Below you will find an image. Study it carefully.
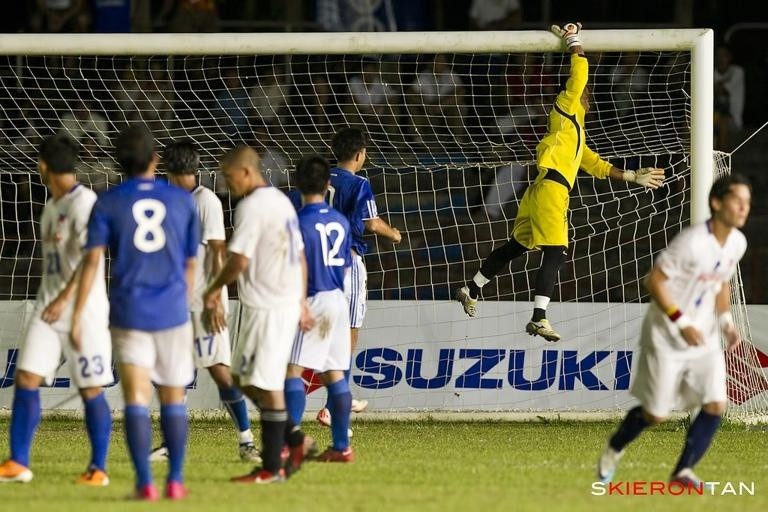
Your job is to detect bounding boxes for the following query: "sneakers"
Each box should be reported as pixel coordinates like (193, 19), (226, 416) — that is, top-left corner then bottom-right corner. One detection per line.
(316, 407), (354, 438)
(0, 459), (34, 484)
(524, 317), (562, 343)
(455, 285), (481, 318)
(667, 469), (709, 490)
(596, 438), (625, 486)
(350, 398), (369, 413)
(238, 441), (264, 465)
(230, 469), (281, 486)
(163, 480), (191, 502)
(280, 432), (317, 480)
(72, 468), (109, 487)
(314, 446), (354, 462)
(148, 446), (170, 462)
(126, 481), (159, 503)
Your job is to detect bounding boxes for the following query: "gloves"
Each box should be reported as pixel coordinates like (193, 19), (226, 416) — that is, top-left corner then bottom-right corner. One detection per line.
(550, 21), (584, 49)
(622, 165), (669, 191)
